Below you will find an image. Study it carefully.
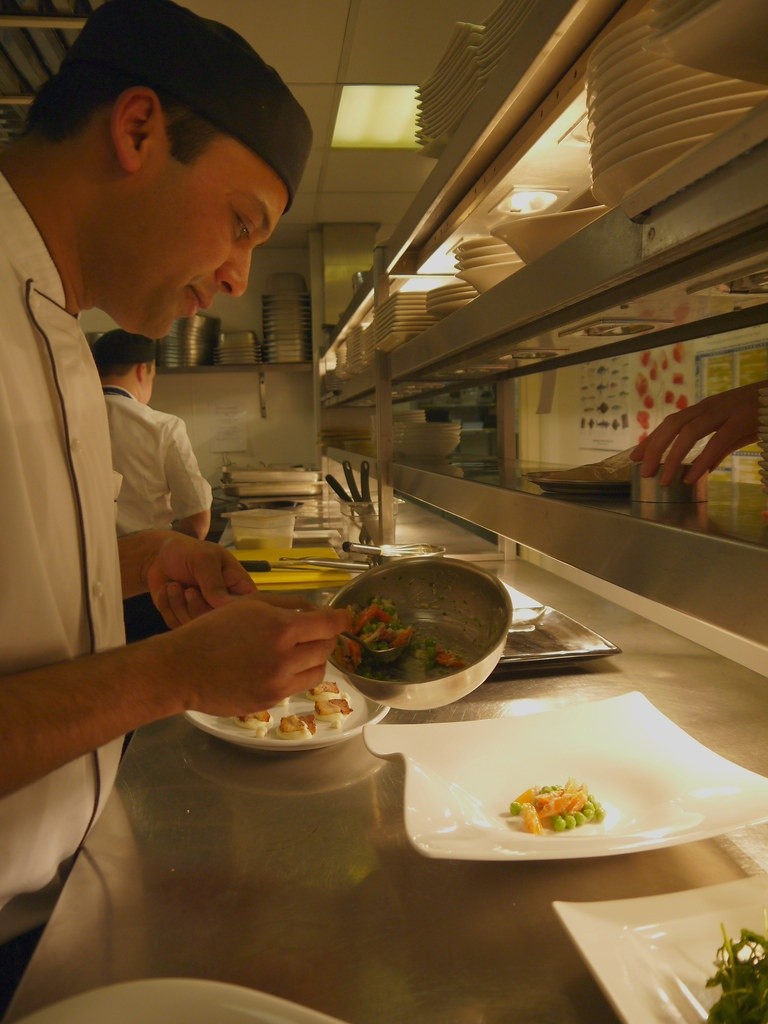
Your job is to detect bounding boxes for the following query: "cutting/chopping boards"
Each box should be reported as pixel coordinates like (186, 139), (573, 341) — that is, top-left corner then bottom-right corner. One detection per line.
(226, 548), (353, 583)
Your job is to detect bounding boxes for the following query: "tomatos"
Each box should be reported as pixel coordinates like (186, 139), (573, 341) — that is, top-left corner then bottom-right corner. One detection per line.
(334, 604), (467, 668)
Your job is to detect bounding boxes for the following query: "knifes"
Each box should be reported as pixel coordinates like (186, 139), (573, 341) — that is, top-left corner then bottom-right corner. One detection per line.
(324, 459), (379, 546)
(239, 560), (307, 572)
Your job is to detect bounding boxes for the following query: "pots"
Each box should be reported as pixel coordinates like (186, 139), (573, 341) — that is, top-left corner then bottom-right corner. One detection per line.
(279, 543), (447, 567)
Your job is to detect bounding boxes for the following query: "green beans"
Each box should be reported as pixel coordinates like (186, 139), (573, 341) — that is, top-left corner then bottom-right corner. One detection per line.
(356, 595), (438, 678)
(510, 786), (606, 831)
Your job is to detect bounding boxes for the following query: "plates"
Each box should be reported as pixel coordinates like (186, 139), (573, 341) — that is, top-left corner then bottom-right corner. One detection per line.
(12, 976), (351, 1024)
(498, 606), (624, 667)
(184, 659), (390, 752)
(503, 581), (544, 609)
(322, 0), (768, 405)
(240, 500), (304, 511)
(525, 470), (633, 494)
(364, 691), (768, 860)
(553, 872), (768, 1024)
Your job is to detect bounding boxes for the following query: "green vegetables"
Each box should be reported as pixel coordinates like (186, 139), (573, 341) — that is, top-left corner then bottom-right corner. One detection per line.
(703, 908), (768, 1024)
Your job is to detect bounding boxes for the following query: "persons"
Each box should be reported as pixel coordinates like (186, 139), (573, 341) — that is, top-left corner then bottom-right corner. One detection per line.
(630, 378), (768, 487)
(0, 0), (354, 1024)
(91, 329), (213, 646)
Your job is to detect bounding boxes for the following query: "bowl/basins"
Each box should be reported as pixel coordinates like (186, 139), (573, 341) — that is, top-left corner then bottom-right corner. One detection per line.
(328, 556), (512, 710)
(370, 409), (497, 461)
(155, 270), (314, 370)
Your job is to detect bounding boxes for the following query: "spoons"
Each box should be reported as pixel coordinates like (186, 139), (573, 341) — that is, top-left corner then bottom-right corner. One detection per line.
(213, 496), (247, 511)
(295, 608), (408, 662)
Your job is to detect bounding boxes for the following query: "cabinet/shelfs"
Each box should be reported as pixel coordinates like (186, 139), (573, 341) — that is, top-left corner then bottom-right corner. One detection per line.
(317, 0), (768, 677)
